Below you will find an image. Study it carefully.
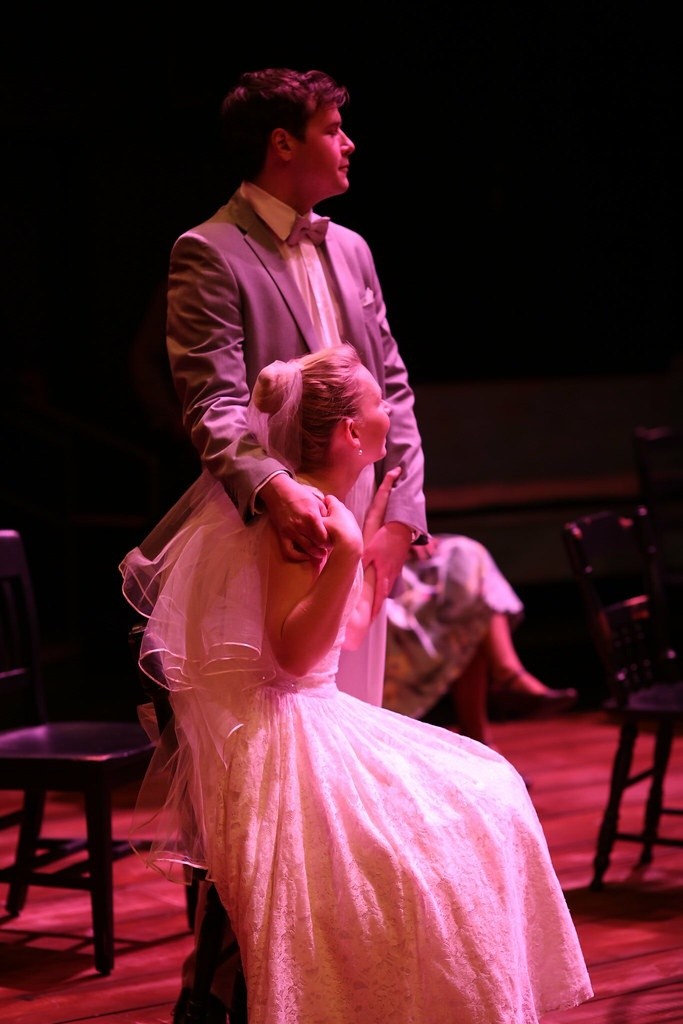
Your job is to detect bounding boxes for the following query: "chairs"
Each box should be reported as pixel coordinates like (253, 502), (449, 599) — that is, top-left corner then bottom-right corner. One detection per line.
(561, 503), (683, 878)
(629, 424), (683, 669)
(127, 620), (247, 1024)
(0, 529), (198, 972)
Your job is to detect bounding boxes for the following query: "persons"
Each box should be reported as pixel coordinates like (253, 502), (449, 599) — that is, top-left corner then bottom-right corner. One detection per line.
(167, 70), (426, 1023)
(115, 340), (595, 1024)
(382, 532), (578, 784)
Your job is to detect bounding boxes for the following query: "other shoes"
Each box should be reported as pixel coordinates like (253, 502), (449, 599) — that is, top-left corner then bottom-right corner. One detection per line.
(486, 675), (575, 721)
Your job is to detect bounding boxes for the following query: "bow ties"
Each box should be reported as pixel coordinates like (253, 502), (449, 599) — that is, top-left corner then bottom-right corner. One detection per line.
(285, 217), (329, 250)
